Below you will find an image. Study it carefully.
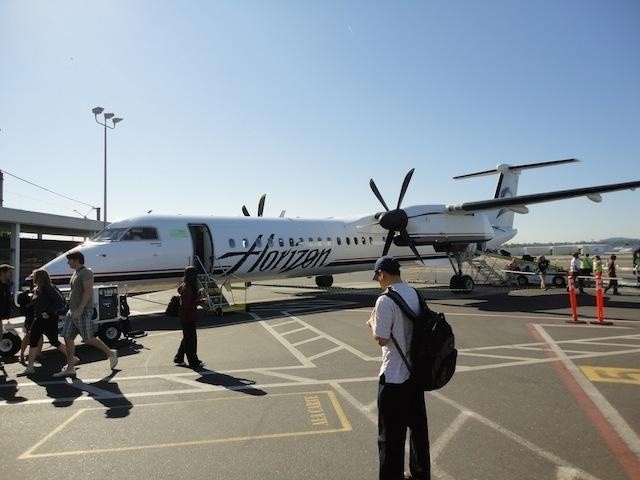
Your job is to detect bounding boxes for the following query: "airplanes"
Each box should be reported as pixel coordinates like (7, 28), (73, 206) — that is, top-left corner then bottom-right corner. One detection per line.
(23, 155), (640, 311)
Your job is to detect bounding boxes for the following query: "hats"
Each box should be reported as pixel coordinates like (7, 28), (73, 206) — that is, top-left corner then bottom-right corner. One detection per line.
(372, 256), (401, 281)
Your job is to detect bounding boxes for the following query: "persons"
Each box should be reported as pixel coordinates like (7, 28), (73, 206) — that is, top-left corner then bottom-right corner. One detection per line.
(507, 258), (520, 289)
(594, 255), (603, 273)
(17, 268), (81, 378)
(17, 274), (48, 366)
(365, 254), (432, 480)
(0, 263), (17, 371)
(172, 265), (207, 368)
(51, 249), (118, 379)
(631, 250), (640, 288)
(566, 252), (585, 293)
(537, 254), (551, 289)
(583, 253), (593, 288)
(604, 254), (621, 295)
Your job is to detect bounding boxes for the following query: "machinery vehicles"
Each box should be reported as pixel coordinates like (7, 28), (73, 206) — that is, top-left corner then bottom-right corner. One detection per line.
(502, 258), (574, 286)
(1, 283), (147, 357)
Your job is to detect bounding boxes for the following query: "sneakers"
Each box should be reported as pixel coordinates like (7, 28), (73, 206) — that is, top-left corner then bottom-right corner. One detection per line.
(539, 288), (622, 296)
(109, 349), (118, 370)
(404, 469), (413, 479)
(173, 358), (205, 369)
(16, 357), (80, 379)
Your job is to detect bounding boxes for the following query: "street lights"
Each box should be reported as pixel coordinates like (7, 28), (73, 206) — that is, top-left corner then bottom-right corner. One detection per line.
(92, 106), (123, 229)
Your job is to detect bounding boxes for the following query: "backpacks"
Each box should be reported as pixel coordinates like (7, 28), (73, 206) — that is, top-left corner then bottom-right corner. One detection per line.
(38, 285), (65, 308)
(374, 286), (458, 392)
